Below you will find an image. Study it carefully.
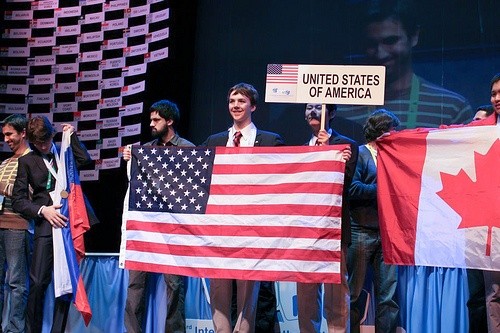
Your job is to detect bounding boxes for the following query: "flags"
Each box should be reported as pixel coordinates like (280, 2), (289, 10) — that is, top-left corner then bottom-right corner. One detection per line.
(49, 128), (93, 328)
(377, 124), (500, 271)
(266, 64), (298, 83)
(117, 144), (349, 286)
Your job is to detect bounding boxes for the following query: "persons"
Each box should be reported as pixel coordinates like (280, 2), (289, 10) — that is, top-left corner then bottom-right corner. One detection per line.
(122, 100), (197, 333)
(485, 79), (500, 331)
(472, 105), (493, 123)
(197, 83), (297, 333)
(351, 109), (404, 333)
(298, 104), (359, 333)
(10, 113), (100, 333)
(336, 0), (473, 135)
(0, 114), (33, 333)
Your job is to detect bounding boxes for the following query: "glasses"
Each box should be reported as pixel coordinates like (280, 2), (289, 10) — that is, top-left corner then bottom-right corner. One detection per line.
(33, 138), (51, 144)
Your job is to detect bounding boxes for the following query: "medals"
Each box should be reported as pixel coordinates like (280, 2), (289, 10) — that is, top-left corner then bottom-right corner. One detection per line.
(60, 190), (69, 199)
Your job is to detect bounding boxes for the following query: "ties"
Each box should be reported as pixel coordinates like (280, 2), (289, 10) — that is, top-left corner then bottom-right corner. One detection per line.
(232, 131), (243, 147)
(315, 139), (322, 146)
